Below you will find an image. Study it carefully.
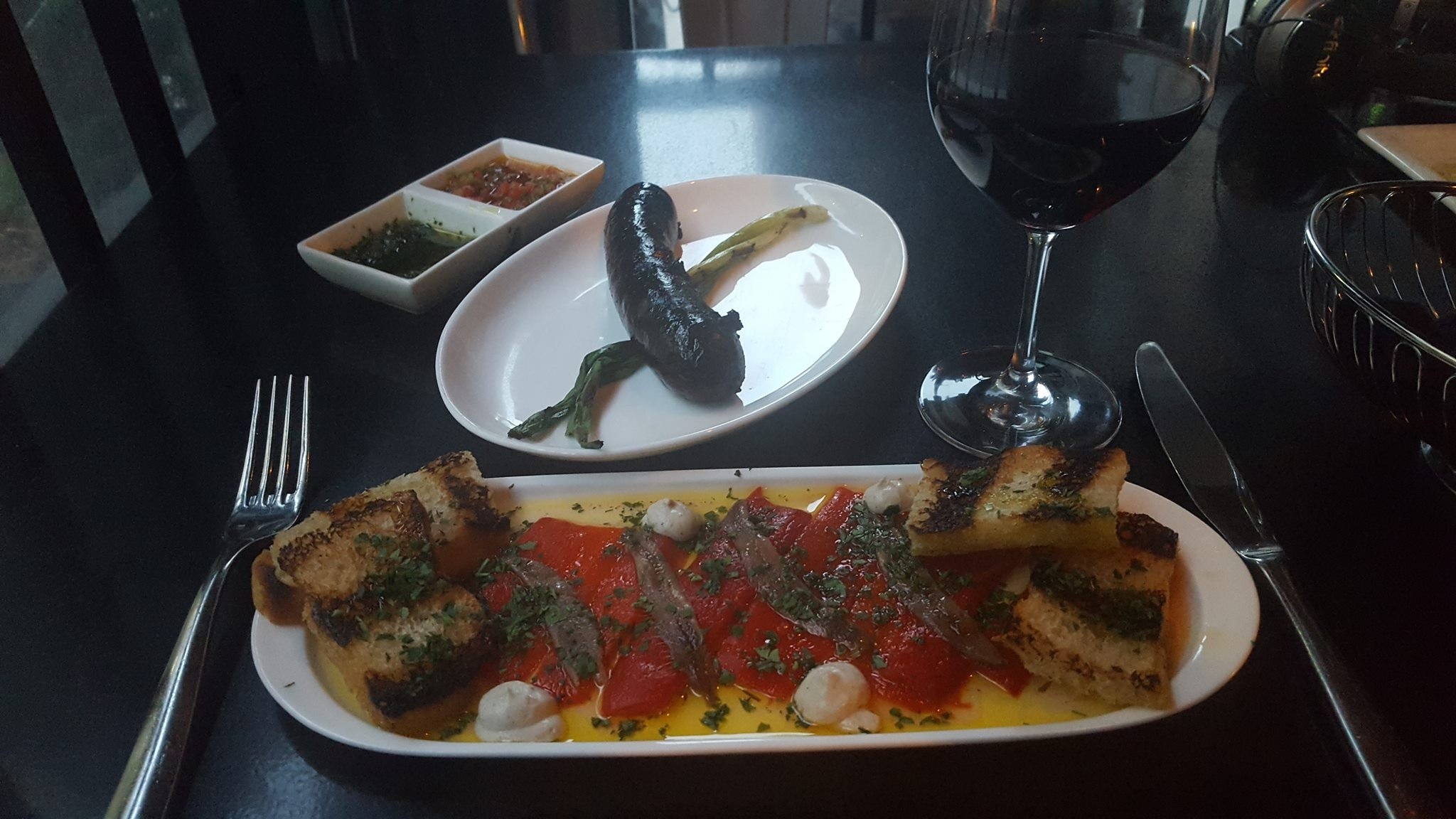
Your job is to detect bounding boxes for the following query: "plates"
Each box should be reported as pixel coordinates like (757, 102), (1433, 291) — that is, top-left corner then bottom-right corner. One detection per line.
(247, 458), (1263, 760)
(434, 172), (905, 461)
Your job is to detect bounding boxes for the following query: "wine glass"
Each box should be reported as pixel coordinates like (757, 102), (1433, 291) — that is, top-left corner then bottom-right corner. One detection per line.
(917, 0), (1230, 460)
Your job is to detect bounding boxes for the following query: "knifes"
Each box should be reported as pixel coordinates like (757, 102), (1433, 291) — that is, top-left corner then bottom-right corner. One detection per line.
(1133, 337), (1450, 819)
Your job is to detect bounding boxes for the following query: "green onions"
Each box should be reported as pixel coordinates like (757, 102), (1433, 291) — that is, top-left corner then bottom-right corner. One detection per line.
(506, 202), (829, 447)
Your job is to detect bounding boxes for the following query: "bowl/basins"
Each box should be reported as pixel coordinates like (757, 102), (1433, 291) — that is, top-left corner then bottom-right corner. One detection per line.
(298, 136), (607, 316)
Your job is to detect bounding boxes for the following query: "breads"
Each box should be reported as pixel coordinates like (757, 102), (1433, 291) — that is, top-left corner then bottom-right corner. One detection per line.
(244, 448), (509, 630)
(895, 440), (1127, 566)
(296, 600), (534, 746)
(1007, 512), (1179, 712)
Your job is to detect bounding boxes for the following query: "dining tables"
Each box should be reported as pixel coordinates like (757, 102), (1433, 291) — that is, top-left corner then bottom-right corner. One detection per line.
(0, 49), (1456, 819)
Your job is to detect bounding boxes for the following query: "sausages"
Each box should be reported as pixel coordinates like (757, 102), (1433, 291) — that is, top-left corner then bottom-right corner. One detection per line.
(604, 176), (748, 402)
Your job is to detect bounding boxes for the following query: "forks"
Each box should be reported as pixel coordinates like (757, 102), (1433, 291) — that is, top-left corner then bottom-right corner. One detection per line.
(95, 369), (312, 819)
